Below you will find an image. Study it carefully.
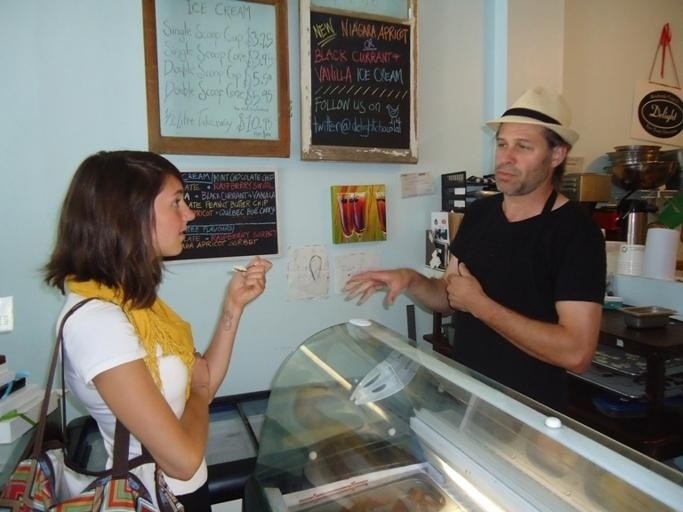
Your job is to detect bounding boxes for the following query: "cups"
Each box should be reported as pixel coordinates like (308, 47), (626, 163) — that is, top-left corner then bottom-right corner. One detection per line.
(337, 193), (387, 242)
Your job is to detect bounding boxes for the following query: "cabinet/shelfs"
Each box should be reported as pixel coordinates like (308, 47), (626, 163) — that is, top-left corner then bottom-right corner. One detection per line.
(243, 320), (683, 512)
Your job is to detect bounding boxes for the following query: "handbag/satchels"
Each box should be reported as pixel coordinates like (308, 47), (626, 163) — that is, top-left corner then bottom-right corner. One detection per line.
(0, 440), (185, 512)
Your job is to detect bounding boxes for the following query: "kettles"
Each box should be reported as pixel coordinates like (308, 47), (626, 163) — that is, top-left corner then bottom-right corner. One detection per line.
(617, 189), (657, 246)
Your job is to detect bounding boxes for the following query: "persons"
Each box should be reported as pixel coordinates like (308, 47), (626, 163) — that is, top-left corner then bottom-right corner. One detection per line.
(40, 149), (274, 512)
(340, 86), (607, 432)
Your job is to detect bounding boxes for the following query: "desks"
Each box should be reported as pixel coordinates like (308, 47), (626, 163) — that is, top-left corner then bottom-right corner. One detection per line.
(423, 305), (683, 463)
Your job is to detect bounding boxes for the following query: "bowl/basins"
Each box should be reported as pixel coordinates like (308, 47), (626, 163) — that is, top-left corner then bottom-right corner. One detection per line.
(603, 146), (681, 190)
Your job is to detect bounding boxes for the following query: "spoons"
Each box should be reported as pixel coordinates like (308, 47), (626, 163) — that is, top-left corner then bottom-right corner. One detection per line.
(233, 264), (256, 273)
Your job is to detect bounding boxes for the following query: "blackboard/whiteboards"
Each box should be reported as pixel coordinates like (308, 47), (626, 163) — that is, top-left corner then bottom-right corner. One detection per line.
(162, 172), (278, 262)
(301, 6), (417, 163)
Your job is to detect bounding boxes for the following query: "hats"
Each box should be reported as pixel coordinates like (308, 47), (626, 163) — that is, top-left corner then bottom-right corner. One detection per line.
(485, 88), (580, 147)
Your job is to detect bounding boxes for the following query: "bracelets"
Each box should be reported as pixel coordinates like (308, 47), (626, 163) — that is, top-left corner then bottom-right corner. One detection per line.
(189, 381), (212, 392)
